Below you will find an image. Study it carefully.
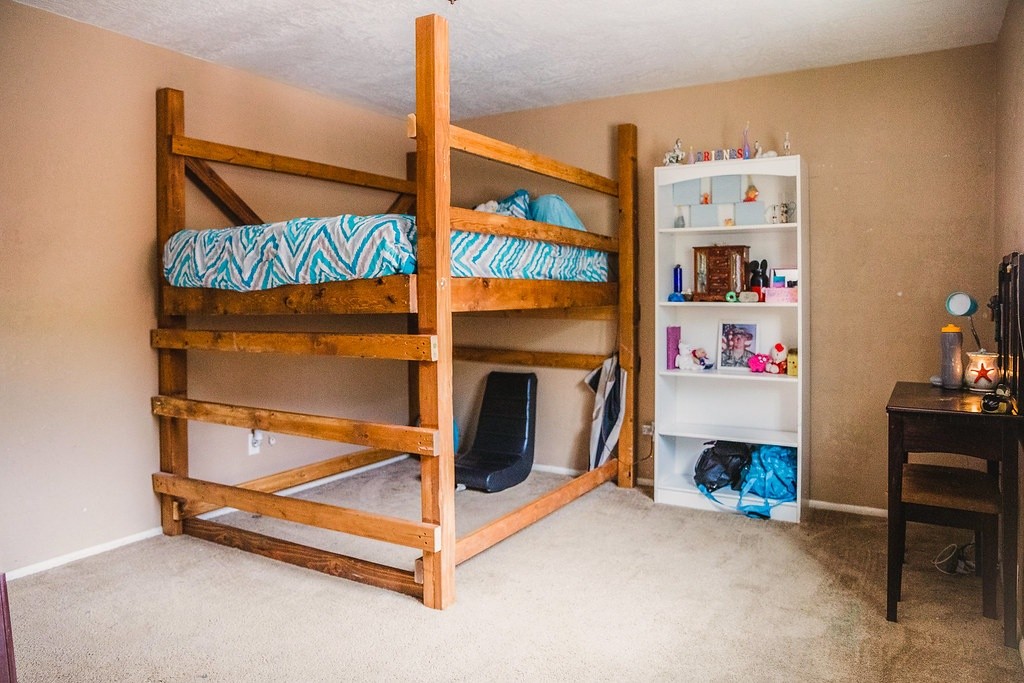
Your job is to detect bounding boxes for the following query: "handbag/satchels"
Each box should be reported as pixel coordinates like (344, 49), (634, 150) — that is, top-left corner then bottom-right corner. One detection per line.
(742, 445), (798, 501)
(694, 440), (752, 492)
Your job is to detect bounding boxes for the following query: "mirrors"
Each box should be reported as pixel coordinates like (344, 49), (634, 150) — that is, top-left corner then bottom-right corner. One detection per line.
(770, 267), (798, 288)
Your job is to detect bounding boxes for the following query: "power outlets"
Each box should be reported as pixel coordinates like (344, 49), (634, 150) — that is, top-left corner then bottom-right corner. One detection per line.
(643, 425), (654, 435)
(250, 426), (261, 454)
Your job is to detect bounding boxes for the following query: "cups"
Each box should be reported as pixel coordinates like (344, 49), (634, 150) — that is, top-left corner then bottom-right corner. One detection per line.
(770, 201), (796, 224)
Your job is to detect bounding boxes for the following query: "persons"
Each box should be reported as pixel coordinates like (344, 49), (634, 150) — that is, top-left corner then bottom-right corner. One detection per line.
(721, 328), (755, 369)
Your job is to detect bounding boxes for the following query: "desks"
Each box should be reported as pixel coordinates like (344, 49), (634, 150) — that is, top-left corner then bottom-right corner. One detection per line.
(885, 379), (1021, 649)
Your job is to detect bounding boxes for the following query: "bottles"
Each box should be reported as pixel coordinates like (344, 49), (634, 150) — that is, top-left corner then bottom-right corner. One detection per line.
(941, 324), (964, 389)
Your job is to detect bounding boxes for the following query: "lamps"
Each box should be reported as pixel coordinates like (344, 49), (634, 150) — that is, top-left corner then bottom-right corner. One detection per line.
(945, 290), (983, 348)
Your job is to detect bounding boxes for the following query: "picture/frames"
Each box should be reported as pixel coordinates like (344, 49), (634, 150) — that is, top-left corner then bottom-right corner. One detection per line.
(717, 321), (762, 369)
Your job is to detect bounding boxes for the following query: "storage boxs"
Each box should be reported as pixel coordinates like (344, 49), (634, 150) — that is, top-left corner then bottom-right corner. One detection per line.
(765, 287), (797, 303)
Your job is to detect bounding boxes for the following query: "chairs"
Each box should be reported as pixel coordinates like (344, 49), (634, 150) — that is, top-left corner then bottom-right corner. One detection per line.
(455, 372), (539, 493)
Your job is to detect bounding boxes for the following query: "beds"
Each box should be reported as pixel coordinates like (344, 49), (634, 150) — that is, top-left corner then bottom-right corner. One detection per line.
(156, 10), (636, 608)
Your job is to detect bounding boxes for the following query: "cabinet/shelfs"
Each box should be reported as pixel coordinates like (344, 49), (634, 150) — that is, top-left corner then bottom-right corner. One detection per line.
(644, 156), (811, 524)
